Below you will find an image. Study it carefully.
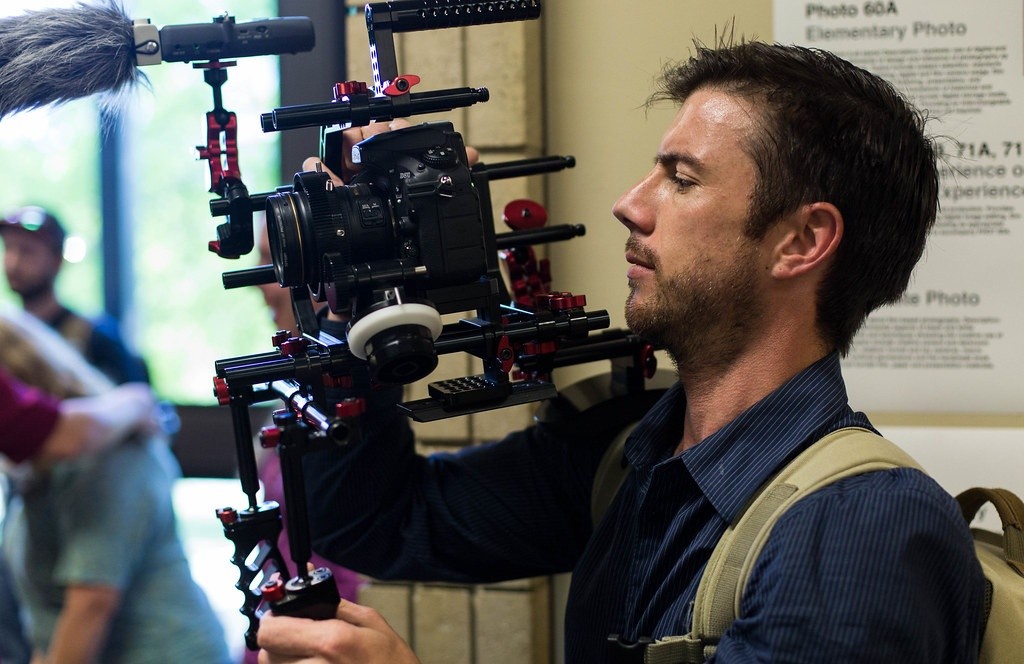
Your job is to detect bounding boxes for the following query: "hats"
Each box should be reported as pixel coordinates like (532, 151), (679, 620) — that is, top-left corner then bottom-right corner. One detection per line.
(0, 205), (64, 255)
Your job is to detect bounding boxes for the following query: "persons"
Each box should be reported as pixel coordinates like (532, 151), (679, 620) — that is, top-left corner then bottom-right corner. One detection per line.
(1, 203), (232, 664)
(239, 215), (358, 664)
(256, 37), (986, 664)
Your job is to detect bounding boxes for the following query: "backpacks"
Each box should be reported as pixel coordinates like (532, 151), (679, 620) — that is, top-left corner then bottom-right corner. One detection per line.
(591, 415), (1024, 664)
